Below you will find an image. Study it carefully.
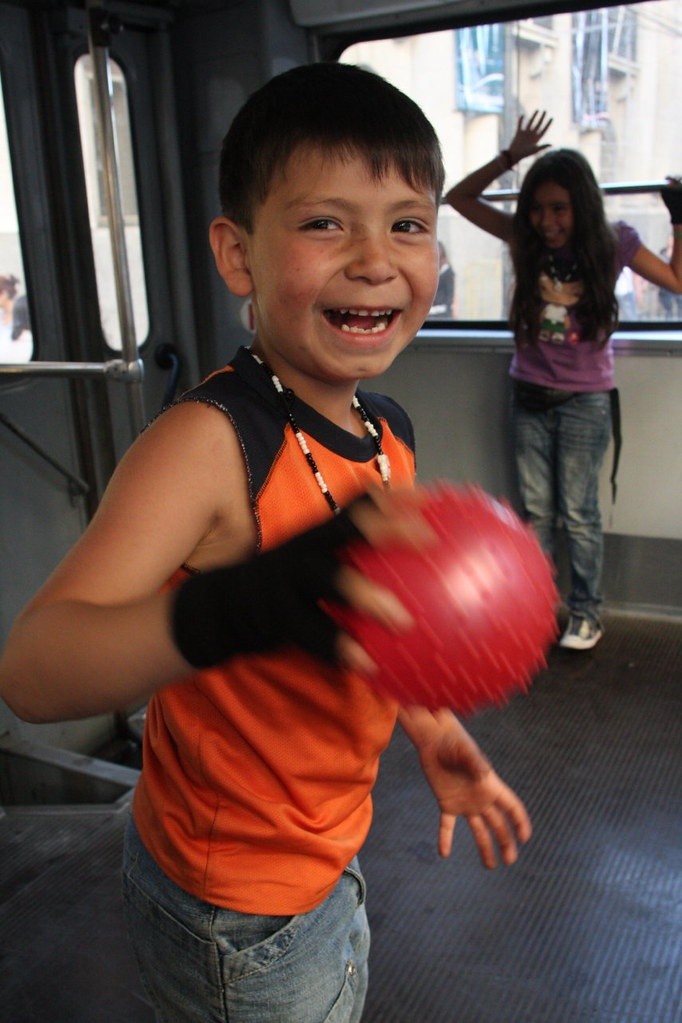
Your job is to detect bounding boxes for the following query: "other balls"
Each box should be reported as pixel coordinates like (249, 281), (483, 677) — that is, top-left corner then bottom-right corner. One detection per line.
(345, 480), (563, 712)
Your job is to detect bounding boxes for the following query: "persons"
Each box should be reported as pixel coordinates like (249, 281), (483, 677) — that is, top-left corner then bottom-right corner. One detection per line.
(0, 274), (36, 360)
(0, 58), (536, 1022)
(445, 107), (682, 652)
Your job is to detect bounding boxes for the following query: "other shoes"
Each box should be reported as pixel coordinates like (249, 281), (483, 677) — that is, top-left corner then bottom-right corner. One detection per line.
(561, 611), (603, 648)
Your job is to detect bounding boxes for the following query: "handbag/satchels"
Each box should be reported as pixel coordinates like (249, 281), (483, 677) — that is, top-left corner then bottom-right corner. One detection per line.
(515, 380), (575, 412)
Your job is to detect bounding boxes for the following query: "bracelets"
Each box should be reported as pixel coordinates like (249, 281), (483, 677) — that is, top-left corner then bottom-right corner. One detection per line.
(499, 150), (513, 170)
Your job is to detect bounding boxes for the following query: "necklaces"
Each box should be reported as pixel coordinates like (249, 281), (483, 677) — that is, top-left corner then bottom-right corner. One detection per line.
(243, 344), (393, 517)
(548, 252), (577, 292)
